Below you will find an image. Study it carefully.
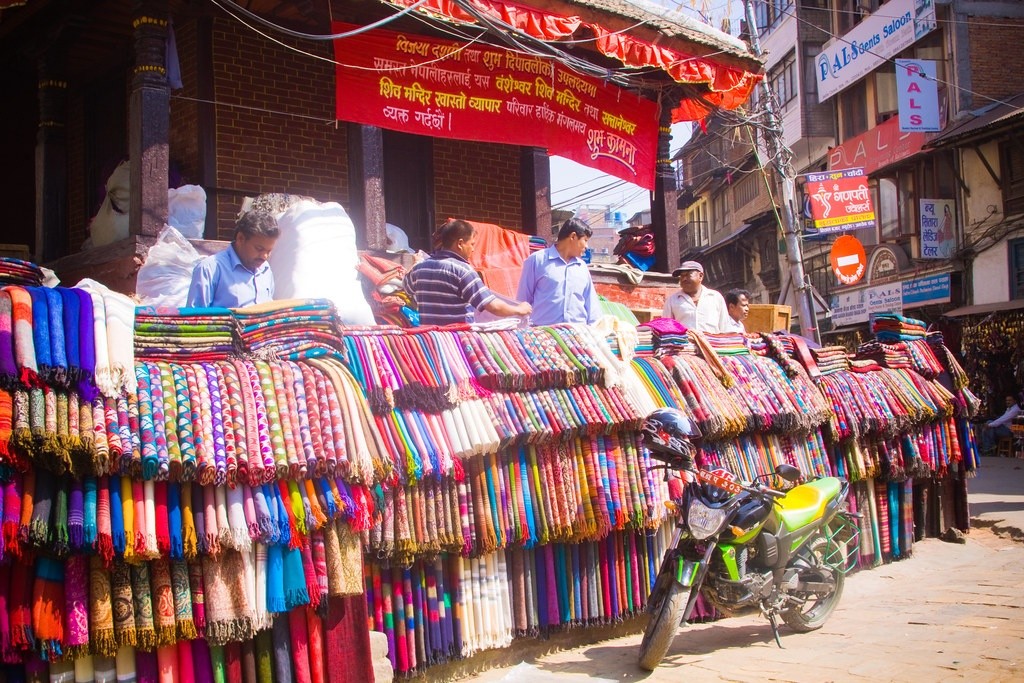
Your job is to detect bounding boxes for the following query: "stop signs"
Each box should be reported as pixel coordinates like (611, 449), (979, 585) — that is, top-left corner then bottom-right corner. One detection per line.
(830, 235), (866, 285)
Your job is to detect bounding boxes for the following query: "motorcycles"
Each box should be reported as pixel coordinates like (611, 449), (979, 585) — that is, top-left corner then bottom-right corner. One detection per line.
(636, 406), (866, 672)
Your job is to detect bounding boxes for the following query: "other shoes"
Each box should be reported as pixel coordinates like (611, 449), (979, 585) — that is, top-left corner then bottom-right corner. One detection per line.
(984, 446), (997, 453)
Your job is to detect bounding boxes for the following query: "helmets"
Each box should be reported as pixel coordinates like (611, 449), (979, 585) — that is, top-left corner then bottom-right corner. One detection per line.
(641, 407), (704, 467)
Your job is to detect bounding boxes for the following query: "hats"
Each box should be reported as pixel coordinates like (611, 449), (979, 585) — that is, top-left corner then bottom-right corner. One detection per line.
(672, 261), (703, 278)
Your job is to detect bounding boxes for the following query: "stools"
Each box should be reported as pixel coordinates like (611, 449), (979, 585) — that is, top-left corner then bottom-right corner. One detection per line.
(998, 437), (1016, 457)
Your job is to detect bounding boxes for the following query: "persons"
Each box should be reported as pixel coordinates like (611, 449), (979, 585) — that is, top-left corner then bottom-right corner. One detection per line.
(724, 287), (750, 333)
(516, 217), (602, 326)
(185, 211), (281, 308)
(402, 219), (533, 326)
(981, 395), (1022, 453)
(662, 261), (733, 334)
(283, 306), (286, 308)
